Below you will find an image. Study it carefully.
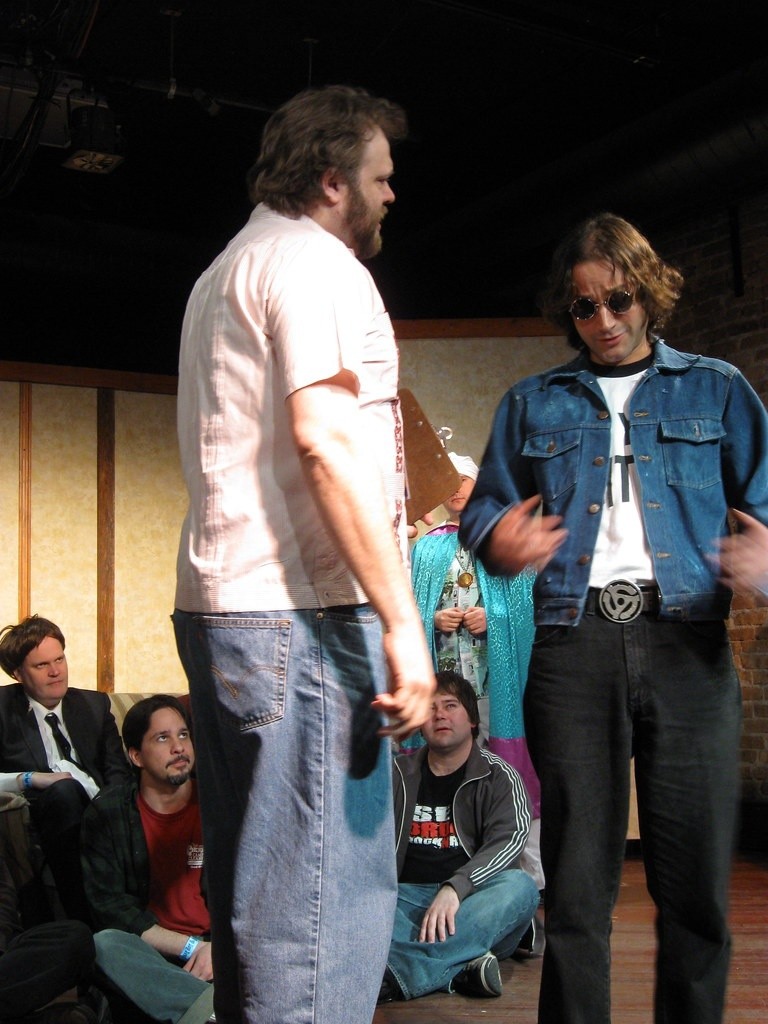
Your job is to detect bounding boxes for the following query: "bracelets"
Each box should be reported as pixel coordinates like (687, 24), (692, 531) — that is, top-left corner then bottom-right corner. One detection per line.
(178, 935), (200, 962)
(22, 771), (35, 789)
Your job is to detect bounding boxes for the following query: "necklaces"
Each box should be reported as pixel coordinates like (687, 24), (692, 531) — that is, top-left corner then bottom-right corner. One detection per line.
(452, 553), (473, 588)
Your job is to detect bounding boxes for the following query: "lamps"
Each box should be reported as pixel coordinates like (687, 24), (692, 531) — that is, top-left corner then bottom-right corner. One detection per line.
(62, 88), (124, 177)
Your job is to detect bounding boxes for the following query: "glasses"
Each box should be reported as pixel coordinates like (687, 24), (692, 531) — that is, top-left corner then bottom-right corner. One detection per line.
(565, 285), (641, 320)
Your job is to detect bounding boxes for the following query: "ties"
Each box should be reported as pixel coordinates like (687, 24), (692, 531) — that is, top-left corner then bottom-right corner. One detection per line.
(45, 713), (89, 773)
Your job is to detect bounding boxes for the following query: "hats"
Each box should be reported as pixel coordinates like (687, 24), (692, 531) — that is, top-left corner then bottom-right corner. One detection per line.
(447, 450), (479, 482)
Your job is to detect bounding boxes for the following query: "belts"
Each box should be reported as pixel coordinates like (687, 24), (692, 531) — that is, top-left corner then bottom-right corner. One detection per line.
(587, 580), (663, 624)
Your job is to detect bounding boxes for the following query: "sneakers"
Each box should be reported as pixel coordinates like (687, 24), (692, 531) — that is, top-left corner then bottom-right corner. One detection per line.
(458, 950), (504, 997)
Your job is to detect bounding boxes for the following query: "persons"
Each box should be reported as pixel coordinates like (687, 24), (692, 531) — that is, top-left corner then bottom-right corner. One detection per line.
(172, 86), (437, 1024)
(456, 214), (768, 1024)
(0, 452), (544, 1024)
